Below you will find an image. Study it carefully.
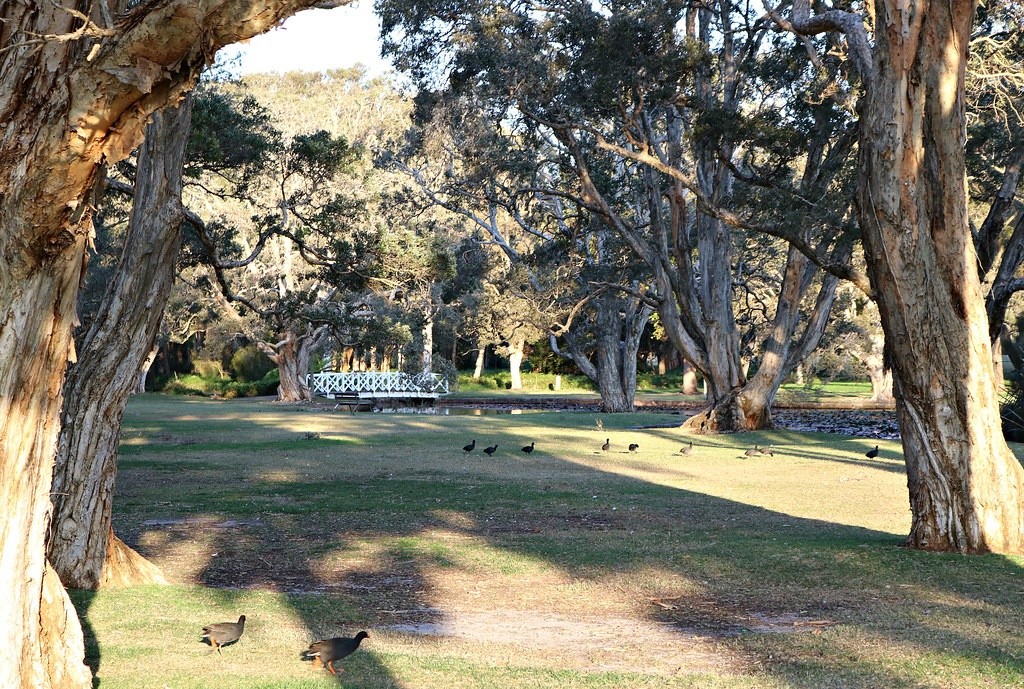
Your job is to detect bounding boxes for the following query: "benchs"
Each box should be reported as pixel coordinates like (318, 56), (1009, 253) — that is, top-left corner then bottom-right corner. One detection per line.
(333, 391), (374, 415)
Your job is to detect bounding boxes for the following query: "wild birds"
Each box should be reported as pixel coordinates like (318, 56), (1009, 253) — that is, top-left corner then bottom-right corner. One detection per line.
(629, 443), (638, 453)
(305, 631), (371, 675)
(202, 614), (246, 657)
(484, 444), (498, 456)
(602, 438), (610, 453)
(680, 441), (693, 454)
(865, 445), (879, 460)
(463, 440), (475, 455)
(521, 442), (535, 455)
(745, 445), (773, 459)
